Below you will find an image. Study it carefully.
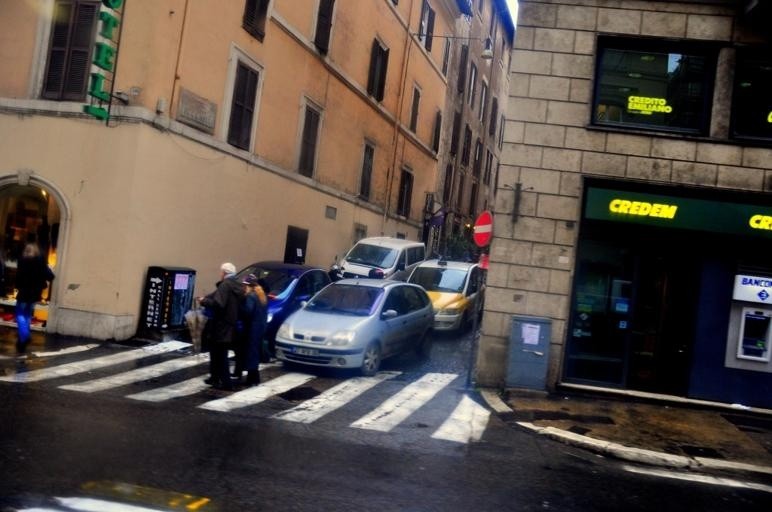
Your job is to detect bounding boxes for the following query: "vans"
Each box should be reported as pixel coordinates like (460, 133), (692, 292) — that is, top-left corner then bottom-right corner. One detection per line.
(338, 236), (427, 281)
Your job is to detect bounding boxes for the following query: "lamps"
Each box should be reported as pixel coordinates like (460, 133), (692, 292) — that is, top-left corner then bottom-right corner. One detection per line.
(411, 32), (494, 58)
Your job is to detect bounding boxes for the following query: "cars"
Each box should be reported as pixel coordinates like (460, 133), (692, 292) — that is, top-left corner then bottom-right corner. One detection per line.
(273, 275), (438, 380)
(193, 259), (335, 362)
(405, 255), (488, 335)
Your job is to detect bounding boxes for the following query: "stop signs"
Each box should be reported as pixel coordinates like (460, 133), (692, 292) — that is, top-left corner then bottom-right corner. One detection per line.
(473, 211), (494, 246)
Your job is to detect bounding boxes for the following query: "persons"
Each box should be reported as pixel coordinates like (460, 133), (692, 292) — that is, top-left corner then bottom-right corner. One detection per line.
(197, 262), (269, 390)
(5, 200), (30, 259)
(13, 242), (56, 353)
(665, 53), (716, 135)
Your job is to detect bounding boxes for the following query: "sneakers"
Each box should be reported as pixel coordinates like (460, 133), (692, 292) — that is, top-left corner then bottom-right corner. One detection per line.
(204, 372), (259, 386)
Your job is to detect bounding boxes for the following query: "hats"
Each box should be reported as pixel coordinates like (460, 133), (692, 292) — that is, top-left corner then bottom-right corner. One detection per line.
(242, 275), (257, 287)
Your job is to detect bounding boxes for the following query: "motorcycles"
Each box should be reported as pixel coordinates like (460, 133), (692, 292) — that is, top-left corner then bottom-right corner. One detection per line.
(326, 253), (384, 284)
